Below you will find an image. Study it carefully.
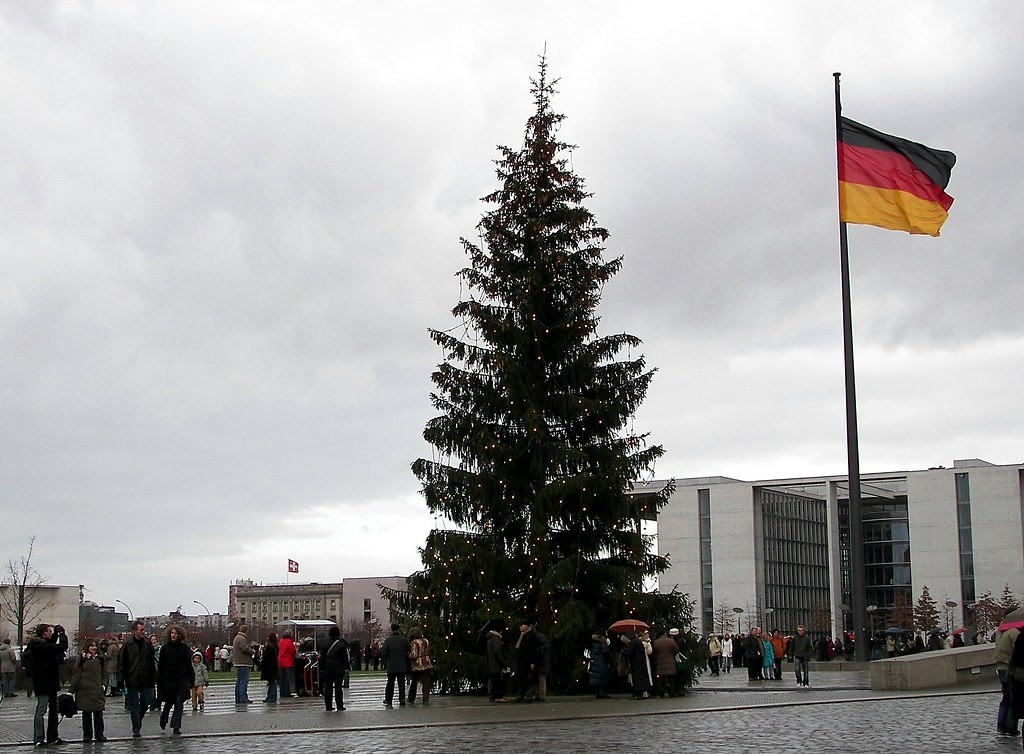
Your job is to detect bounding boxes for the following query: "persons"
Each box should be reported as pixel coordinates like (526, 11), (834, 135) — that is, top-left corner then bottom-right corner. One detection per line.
(277, 629), (296, 698)
(260, 632), (279, 703)
(381, 623), (411, 705)
(590, 626), (685, 699)
(487, 617), (549, 704)
(788, 625), (813, 688)
(319, 626), (351, 712)
(0, 633), (360, 713)
(233, 625), (257, 704)
(694, 626), (842, 681)
(68, 637), (109, 743)
(360, 638), (389, 672)
(995, 605), (1024, 737)
(30, 624), (68, 747)
(157, 624), (195, 735)
(843, 629), (996, 664)
(408, 627), (434, 706)
(117, 620), (157, 739)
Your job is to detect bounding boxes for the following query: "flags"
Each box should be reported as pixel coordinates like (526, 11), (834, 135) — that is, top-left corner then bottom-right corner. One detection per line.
(837, 115), (956, 238)
(289, 559), (299, 573)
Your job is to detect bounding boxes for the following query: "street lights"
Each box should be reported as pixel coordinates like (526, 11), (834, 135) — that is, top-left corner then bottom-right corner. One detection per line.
(945, 601), (957, 629)
(733, 608), (744, 635)
(838, 605), (850, 631)
(764, 609), (774, 633)
(369, 619), (378, 643)
(115, 599), (133, 624)
(867, 605), (877, 637)
(193, 600), (213, 642)
(332, 603), (350, 645)
(226, 622), (235, 644)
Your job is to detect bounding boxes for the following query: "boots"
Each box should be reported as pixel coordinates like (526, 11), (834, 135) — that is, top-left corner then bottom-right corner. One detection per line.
(106, 686), (117, 697)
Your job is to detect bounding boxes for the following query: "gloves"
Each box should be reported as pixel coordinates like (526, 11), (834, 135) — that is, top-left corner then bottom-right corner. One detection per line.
(205, 682), (209, 687)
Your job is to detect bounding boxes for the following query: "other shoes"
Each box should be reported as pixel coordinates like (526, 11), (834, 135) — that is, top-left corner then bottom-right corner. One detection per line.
(326, 708), (335, 712)
(383, 700), (392, 706)
(34, 740), (48, 748)
(83, 739), (93, 745)
(160, 721), (166, 730)
(95, 734), (107, 742)
(200, 703), (204, 712)
(173, 727), (183, 734)
(797, 683), (801, 687)
(47, 737), (67, 746)
(337, 707), (346, 711)
(133, 732), (141, 737)
(996, 728), (1022, 738)
(406, 698), (414, 703)
(236, 699), (253, 704)
(263, 697), (276, 702)
(193, 707), (197, 711)
(805, 684), (810, 688)
(495, 698), (508, 703)
(4, 692), (17, 697)
